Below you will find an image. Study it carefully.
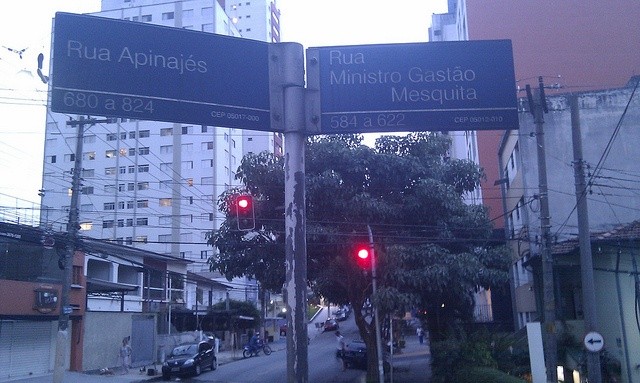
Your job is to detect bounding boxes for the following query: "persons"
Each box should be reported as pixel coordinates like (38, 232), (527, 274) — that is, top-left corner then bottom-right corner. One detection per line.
(117, 339), (133, 375)
(334, 329), (349, 372)
(416, 324), (425, 344)
(249, 331), (264, 356)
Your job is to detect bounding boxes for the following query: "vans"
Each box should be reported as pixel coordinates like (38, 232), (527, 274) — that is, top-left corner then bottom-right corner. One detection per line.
(342, 328), (370, 369)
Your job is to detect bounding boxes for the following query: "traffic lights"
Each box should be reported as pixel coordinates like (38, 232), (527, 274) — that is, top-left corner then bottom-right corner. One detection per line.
(238, 197), (252, 226)
(358, 247), (369, 265)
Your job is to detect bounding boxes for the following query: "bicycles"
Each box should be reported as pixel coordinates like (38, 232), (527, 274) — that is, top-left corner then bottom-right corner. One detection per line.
(383, 355), (390, 374)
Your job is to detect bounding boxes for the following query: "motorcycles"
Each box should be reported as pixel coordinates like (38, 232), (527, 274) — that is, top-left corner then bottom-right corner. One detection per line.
(242, 337), (272, 358)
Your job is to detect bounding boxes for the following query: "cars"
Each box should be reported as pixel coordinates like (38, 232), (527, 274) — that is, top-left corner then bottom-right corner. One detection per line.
(336, 308), (348, 320)
(280, 318), (288, 335)
(323, 318), (340, 331)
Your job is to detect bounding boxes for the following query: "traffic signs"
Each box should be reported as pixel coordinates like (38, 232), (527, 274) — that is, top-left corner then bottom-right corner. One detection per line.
(305, 37), (522, 135)
(49, 10), (285, 132)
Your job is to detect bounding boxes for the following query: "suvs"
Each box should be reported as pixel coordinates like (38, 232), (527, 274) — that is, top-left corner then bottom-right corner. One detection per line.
(161, 340), (217, 379)
